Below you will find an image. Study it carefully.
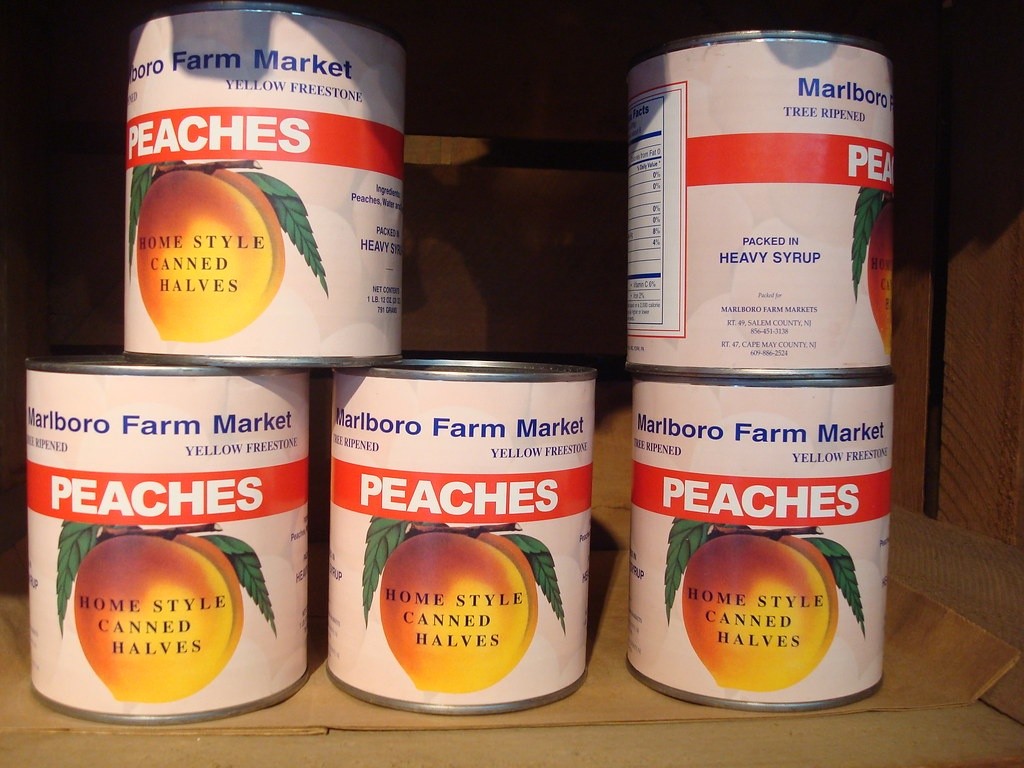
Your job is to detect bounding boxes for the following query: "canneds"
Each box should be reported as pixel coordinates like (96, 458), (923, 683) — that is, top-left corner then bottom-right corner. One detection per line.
(622, 31), (898, 713)
(24, 354), (311, 729)
(123, 0), (413, 369)
(323, 355), (601, 715)
